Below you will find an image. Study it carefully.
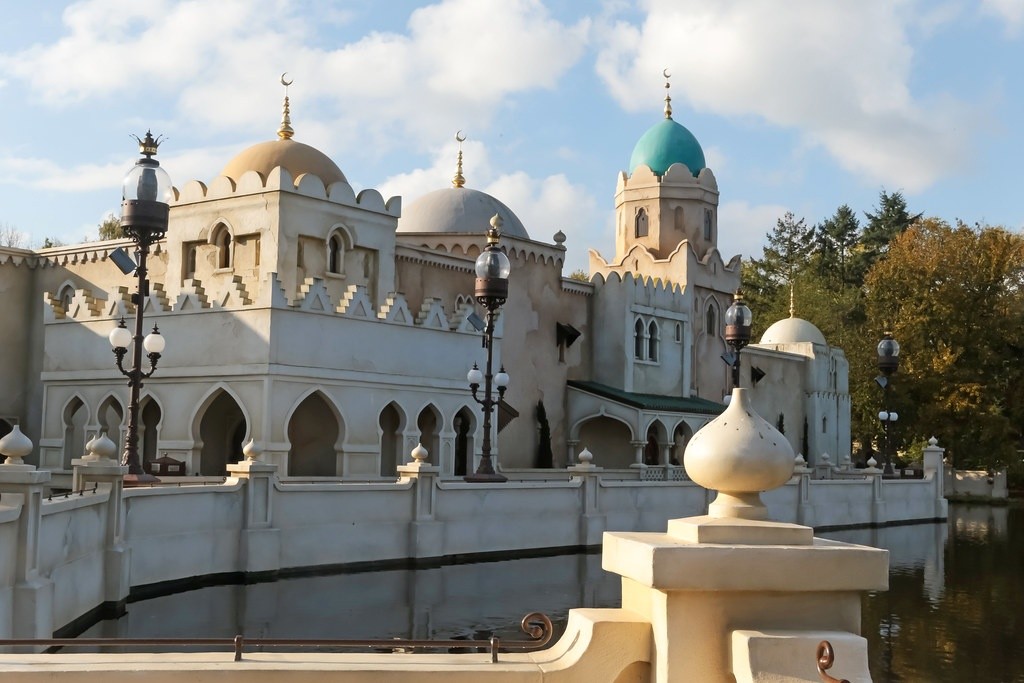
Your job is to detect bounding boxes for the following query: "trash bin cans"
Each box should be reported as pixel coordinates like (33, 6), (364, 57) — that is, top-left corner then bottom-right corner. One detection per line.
(147, 457), (186, 476)
(901, 466), (923, 479)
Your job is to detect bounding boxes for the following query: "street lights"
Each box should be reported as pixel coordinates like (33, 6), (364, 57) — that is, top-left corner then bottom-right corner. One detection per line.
(717, 284), (757, 408)
(108, 128), (177, 487)
(874, 318), (903, 480)
(461, 209), (510, 483)
